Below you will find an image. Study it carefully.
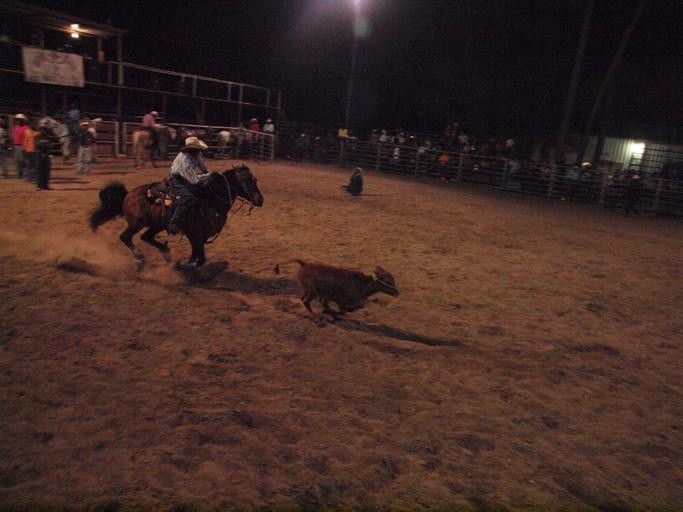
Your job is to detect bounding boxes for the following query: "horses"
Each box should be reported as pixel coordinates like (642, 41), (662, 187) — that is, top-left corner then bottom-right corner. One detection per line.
(216, 131), (230, 155)
(37, 115), (96, 163)
(87, 163), (264, 271)
(132, 127), (176, 157)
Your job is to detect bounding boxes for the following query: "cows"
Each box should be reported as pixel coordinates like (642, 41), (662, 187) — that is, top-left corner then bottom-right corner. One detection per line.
(275, 259), (399, 323)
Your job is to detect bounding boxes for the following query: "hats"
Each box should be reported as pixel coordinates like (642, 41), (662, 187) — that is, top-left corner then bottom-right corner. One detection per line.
(13, 112), (28, 120)
(182, 136), (209, 151)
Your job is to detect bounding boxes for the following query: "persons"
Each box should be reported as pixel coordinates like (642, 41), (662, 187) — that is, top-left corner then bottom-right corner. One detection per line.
(282, 127), (336, 164)
(520, 153), (683, 216)
(411, 123), (515, 185)
(140, 110), (163, 151)
(0, 101), (105, 192)
(337, 125), (410, 171)
(345, 167), (364, 195)
(215, 118), (276, 148)
(165, 136), (215, 235)
